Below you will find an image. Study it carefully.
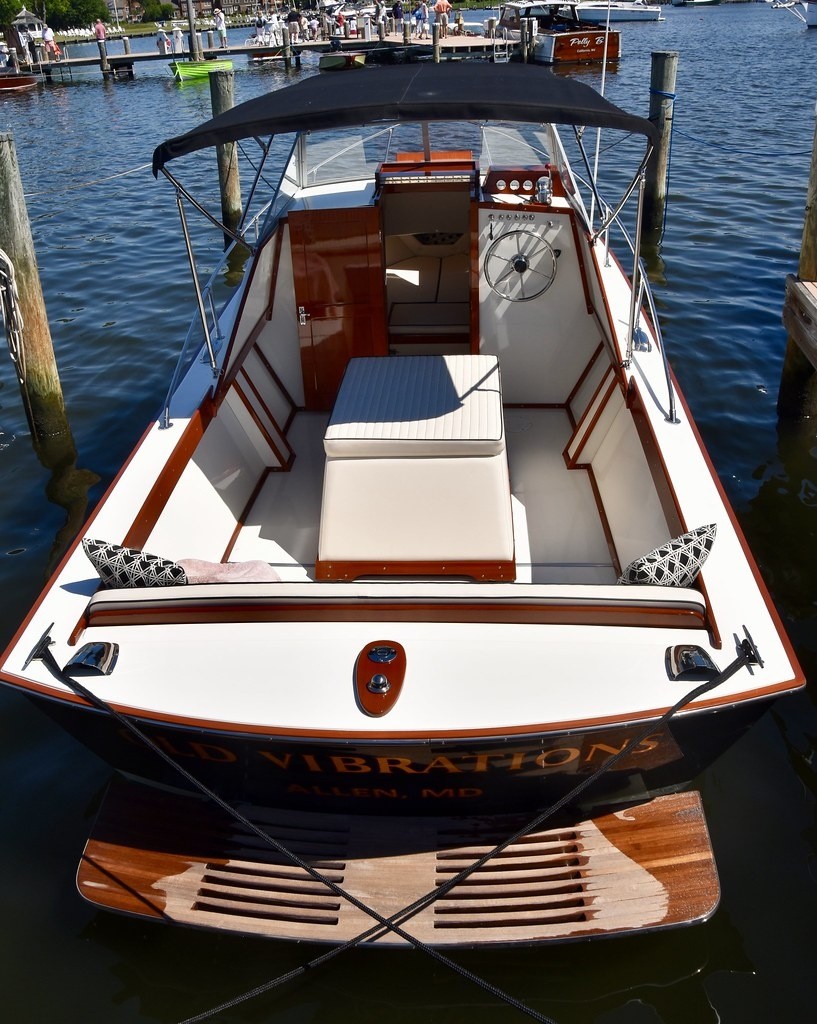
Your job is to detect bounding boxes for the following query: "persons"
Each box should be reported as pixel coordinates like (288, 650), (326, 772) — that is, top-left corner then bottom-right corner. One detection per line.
(213, 8), (228, 48)
(42, 24), (56, 60)
(455, 9), (464, 29)
(156, 27), (184, 51)
(412, 0), (452, 40)
(95, 19), (108, 57)
(23, 25), (38, 64)
(255, 0), (405, 44)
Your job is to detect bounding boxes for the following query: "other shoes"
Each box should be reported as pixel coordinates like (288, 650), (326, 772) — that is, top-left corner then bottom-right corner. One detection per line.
(294, 42), (298, 44)
(225, 46), (227, 48)
(419, 36), (422, 39)
(395, 34), (397, 36)
(386, 34), (389, 36)
(219, 46), (224, 48)
(426, 37), (430, 39)
(290, 42), (292, 44)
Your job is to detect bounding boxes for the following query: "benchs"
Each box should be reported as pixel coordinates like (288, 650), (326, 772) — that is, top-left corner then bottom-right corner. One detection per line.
(87, 580), (707, 629)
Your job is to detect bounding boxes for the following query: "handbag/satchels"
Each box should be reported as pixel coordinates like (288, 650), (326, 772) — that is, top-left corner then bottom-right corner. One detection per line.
(423, 21), (429, 31)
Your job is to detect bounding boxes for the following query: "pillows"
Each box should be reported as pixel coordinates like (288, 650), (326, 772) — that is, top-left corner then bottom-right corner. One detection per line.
(82, 537), (189, 589)
(176, 558), (280, 584)
(616, 523), (717, 585)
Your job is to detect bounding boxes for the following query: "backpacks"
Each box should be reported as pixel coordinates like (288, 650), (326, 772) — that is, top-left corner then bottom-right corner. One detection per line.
(257, 18), (263, 27)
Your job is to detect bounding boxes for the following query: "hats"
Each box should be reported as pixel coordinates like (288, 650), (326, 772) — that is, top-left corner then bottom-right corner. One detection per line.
(213, 8), (221, 14)
(291, 8), (296, 12)
(43, 24), (47, 29)
(268, 8), (274, 13)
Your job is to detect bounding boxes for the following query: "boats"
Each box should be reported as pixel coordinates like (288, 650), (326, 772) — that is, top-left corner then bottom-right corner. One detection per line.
(492, 0), (620, 65)
(0, 65), (38, 94)
(165, 60), (234, 82)
(550, 0), (667, 20)
(172, 76), (210, 98)
(0, 53), (809, 951)
